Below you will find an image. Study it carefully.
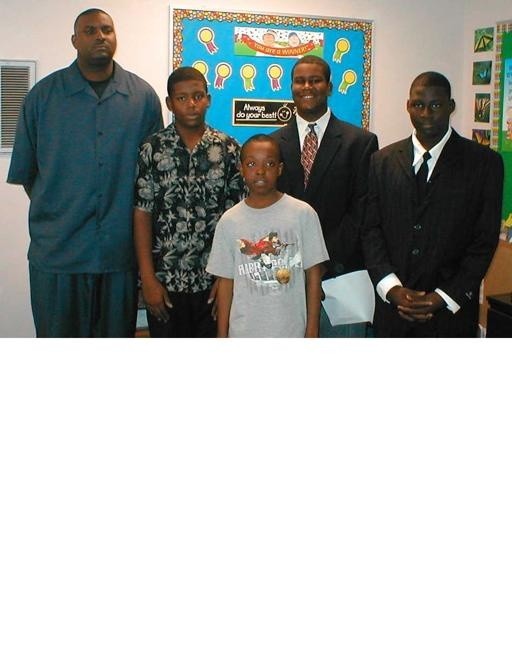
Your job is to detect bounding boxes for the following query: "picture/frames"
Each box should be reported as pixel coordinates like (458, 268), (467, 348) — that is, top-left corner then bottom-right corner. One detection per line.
(168, 4), (377, 148)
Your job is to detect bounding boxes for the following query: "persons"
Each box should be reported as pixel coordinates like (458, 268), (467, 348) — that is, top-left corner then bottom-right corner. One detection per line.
(203, 134), (332, 337)
(5, 6), (168, 337)
(264, 54), (379, 338)
(129, 64), (248, 337)
(363, 67), (505, 338)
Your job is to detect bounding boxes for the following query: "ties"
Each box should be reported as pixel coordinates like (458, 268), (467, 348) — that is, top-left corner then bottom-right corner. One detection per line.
(300, 124), (318, 191)
(414, 151), (432, 181)
